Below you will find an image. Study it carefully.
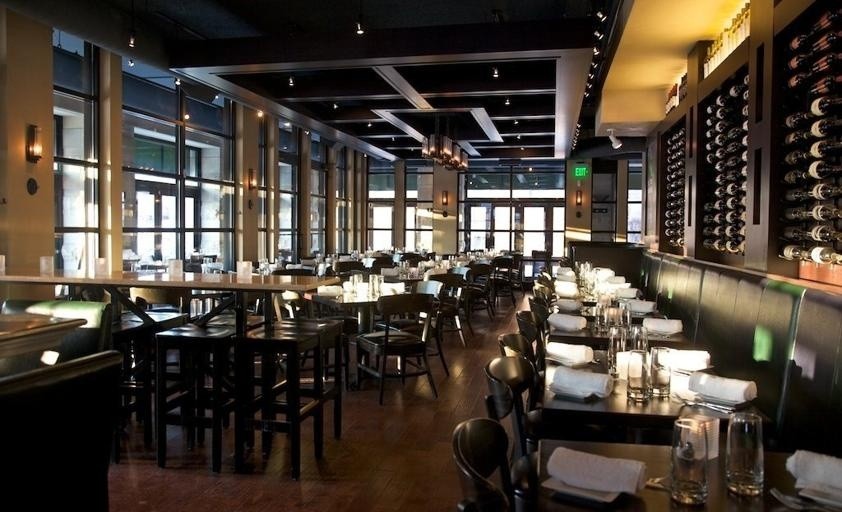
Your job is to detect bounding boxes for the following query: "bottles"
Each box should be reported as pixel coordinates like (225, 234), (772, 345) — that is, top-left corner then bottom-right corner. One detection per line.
(809, 203), (841, 222)
(809, 31), (842, 54)
(807, 160), (841, 180)
(808, 183), (842, 201)
(787, 70), (812, 90)
(784, 129), (813, 146)
(787, 30), (814, 52)
(810, 95), (842, 116)
(784, 112), (814, 130)
(784, 207), (813, 221)
(703, 2), (752, 79)
(806, 8), (842, 38)
(808, 52), (840, 75)
(808, 139), (842, 159)
(785, 188), (812, 201)
(808, 245), (842, 265)
(573, 254), (593, 287)
(785, 51), (813, 73)
(665, 72), (688, 115)
(776, 245), (813, 261)
(785, 149), (813, 165)
(781, 226), (816, 241)
(664, 127), (686, 248)
(701, 75), (749, 255)
(807, 74), (842, 96)
(784, 169), (810, 184)
(806, 223), (841, 243)
(810, 119), (842, 138)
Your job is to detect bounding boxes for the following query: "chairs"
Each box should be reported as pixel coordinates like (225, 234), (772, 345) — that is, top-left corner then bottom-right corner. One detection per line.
(433, 285), (468, 347)
(373, 260), (393, 268)
(429, 273), (476, 337)
(333, 260), (364, 276)
(461, 270), (497, 322)
(528, 295), (553, 336)
(497, 332), (545, 412)
(271, 293), (358, 381)
(538, 274), (556, 291)
(463, 256), (517, 307)
(2, 298), (113, 351)
(375, 279), (451, 378)
(483, 356), (543, 457)
(534, 278), (556, 296)
(491, 253), (525, 296)
(449, 418), (537, 512)
(516, 310), (550, 358)
(532, 286), (556, 307)
(355, 293), (438, 405)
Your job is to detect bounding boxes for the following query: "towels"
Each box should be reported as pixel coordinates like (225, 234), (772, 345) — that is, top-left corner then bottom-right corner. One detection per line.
(545, 342), (593, 365)
(616, 287), (637, 298)
(547, 313), (587, 332)
(793, 449), (842, 493)
(557, 299), (578, 311)
(548, 447), (647, 498)
(383, 268), (398, 276)
(627, 300), (657, 314)
(553, 365), (615, 398)
(557, 274), (576, 283)
(642, 317), (683, 335)
(286, 264), (301, 269)
(422, 260), (434, 267)
(558, 288), (578, 298)
(657, 349), (711, 372)
(301, 260), (315, 266)
(689, 370), (758, 404)
(607, 276), (626, 284)
(318, 286), (343, 296)
(343, 281), (353, 293)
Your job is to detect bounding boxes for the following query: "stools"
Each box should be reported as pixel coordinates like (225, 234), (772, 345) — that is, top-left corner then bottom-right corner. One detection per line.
(230, 326), (324, 480)
(154, 324), (236, 476)
(205, 314), (266, 429)
(109, 321), (152, 465)
(123, 312), (189, 445)
(269, 319), (342, 461)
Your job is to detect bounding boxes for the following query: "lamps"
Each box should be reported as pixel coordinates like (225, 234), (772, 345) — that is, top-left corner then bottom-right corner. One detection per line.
(606, 128), (623, 149)
(576, 191), (581, 204)
(250, 170), (257, 189)
(442, 191), (447, 203)
(33, 128), (43, 160)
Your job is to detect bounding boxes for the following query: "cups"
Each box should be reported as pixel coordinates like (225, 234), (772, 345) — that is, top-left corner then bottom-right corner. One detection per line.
(131, 263), (141, 271)
(620, 303), (632, 331)
(726, 412), (766, 498)
(259, 258), (268, 275)
(368, 275), (379, 298)
(417, 263), (425, 274)
(475, 252), (480, 260)
(668, 418), (709, 508)
(406, 260), (410, 268)
(630, 324), (649, 351)
(650, 347), (671, 397)
(435, 255), (442, 266)
(203, 258), (212, 273)
(449, 255), (457, 267)
(609, 326), (627, 365)
(399, 261), (405, 271)
(354, 274), (363, 291)
(375, 276), (384, 296)
(627, 349), (652, 401)
(467, 252), (472, 259)
(596, 303), (609, 331)
(484, 248), (488, 255)
